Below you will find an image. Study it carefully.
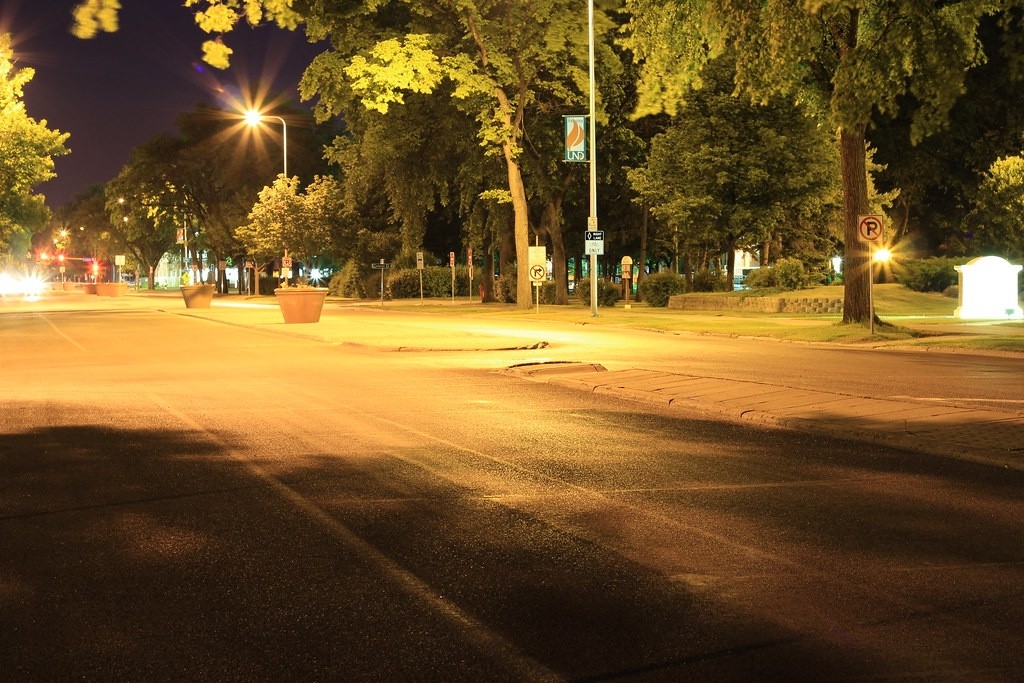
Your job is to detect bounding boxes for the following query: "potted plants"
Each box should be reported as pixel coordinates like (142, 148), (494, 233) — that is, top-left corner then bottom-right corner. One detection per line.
(275, 281), (329, 323)
(50, 280), (128, 296)
(179, 281), (217, 310)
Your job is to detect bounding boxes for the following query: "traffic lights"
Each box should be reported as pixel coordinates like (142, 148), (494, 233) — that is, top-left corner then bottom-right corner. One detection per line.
(93, 263), (98, 272)
(58, 254), (65, 261)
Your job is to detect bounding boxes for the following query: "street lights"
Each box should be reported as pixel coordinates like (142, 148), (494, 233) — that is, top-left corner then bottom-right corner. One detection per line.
(248, 112), (288, 286)
